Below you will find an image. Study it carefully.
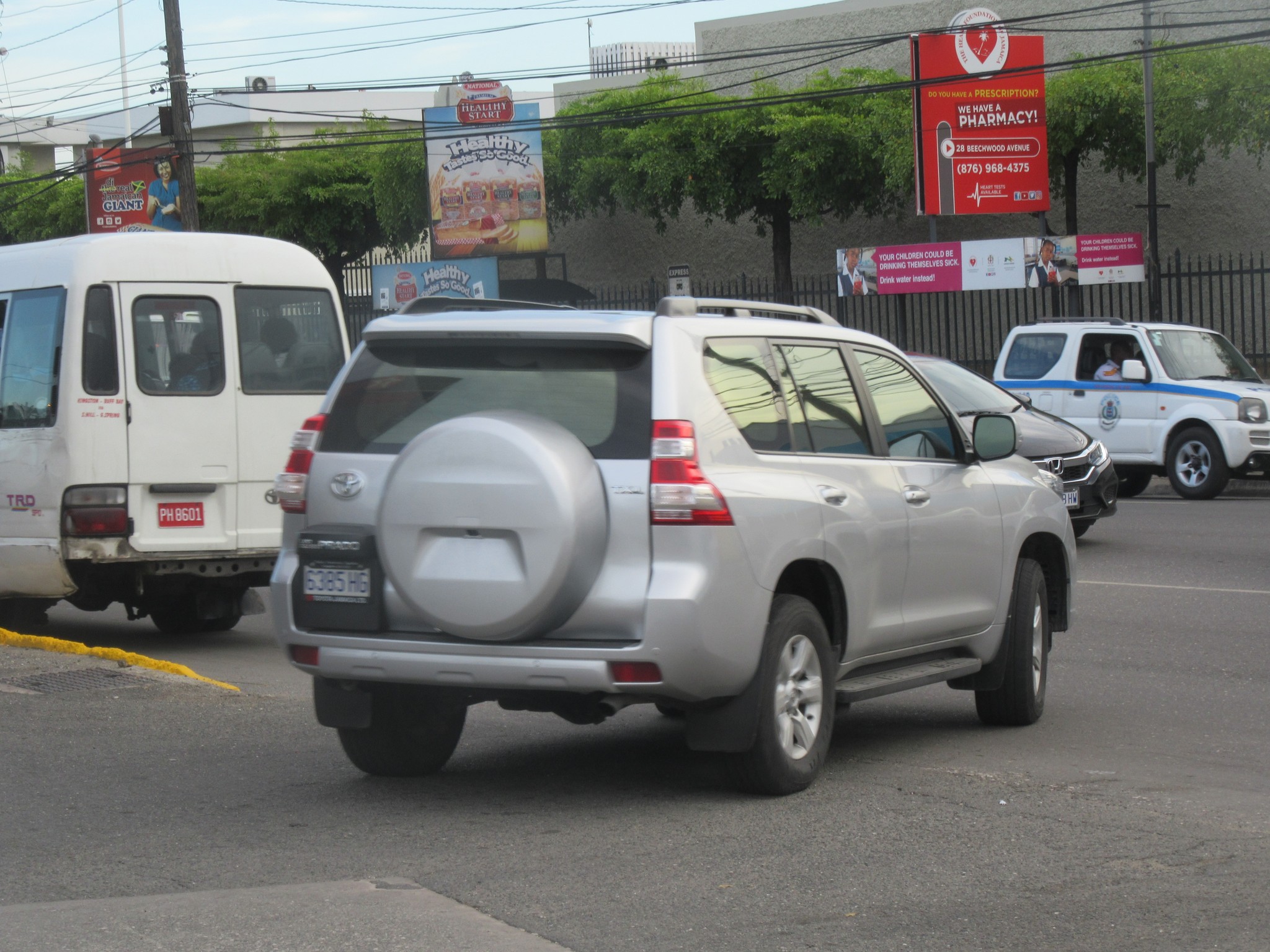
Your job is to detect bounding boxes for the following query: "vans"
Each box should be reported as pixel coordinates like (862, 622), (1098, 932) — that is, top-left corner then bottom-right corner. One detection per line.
(0, 232), (350, 636)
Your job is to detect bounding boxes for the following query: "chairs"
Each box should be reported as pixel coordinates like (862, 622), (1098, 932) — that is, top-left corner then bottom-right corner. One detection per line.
(1077, 345), (1108, 380)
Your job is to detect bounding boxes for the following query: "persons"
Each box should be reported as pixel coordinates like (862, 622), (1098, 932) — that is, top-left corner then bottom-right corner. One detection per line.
(1029, 238), (1061, 287)
(1093, 339), (1144, 384)
(837, 248), (868, 295)
(146, 155), (181, 232)
(80, 306), (332, 399)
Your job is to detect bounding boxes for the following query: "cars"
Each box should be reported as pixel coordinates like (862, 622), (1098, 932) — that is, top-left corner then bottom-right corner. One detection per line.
(900, 350), (1118, 540)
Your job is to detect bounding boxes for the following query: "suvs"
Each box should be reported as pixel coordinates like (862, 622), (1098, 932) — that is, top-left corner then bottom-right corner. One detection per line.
(264, 291), (1084, 798)
(993, 315), (1269, 500)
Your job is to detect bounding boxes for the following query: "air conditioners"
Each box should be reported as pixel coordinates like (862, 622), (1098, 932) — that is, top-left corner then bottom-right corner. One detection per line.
(644, 56), (681, 72)
(245, 76), (276, 92)
